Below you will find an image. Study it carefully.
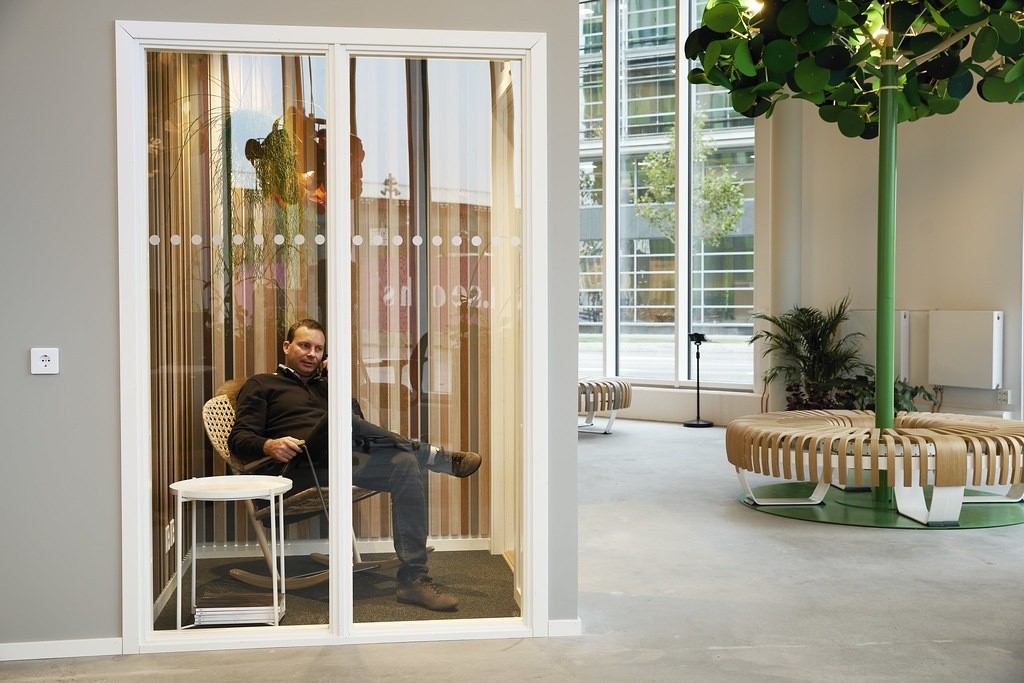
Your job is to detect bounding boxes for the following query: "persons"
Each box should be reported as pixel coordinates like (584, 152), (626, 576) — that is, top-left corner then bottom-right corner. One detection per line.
(226, 319), (482, 610)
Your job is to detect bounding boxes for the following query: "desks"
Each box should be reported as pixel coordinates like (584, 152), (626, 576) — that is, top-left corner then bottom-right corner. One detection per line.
(170, 475), (293, 630)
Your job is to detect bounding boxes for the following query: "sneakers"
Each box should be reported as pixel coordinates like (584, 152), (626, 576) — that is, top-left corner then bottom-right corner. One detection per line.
(427, 446), (482, 477)
(398, 575), (459, 613)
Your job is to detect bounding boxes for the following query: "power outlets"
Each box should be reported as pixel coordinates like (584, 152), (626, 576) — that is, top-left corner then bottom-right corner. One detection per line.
(31, 348), (60, 376)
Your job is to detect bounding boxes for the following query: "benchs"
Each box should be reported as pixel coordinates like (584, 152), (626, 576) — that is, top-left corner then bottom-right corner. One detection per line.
(578, 376), (633, 435)
(726, 410), (1024, 530)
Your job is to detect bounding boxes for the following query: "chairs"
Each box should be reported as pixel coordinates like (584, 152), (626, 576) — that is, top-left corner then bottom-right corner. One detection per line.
(202, 396), (435, 590)
